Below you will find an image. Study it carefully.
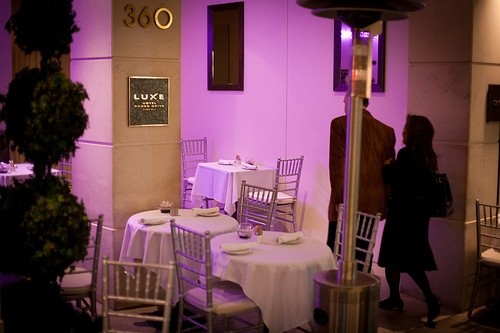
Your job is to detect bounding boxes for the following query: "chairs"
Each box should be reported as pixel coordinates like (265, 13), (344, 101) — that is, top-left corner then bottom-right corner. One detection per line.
(467, 199), (500, 330)
(180, 137), (221, 210)
(333, 203), (380, 273)
(57, 214), (104, 316)
(102, 255), (173, 333)
(249, 155), (305, 232)
(239, 180), (278, 232)
(170, 219), (262, 333)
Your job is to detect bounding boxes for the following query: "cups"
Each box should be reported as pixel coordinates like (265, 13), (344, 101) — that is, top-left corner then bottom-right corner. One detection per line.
(170, 200), (178, 216)
(159, 200), (171, 213)
(237, 223), (254, 239)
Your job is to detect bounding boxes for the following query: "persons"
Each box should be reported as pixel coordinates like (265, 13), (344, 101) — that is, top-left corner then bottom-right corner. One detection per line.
(377, 112), (442, 324)
(324, 90), (398, 273)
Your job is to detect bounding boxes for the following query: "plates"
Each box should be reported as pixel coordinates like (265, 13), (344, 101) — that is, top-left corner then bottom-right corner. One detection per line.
(243, 167), (256, 170)
(139, 220), (165, 224)
(199, 209), (219, 216)
(218, 162), (234, 165)
(222, 243), (252, 254)
(277, 232), (304, 244)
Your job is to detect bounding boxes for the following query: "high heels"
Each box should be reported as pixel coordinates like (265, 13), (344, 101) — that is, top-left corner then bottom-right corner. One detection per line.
(379, 296), (404, 312)
(425, 295), (441, 321)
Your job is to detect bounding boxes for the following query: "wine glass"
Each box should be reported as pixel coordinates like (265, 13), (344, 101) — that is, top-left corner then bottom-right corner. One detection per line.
(254, 224), (264, 243)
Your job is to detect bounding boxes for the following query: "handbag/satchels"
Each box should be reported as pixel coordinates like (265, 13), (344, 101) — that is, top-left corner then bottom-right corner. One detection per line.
(430, 170), (454, 217)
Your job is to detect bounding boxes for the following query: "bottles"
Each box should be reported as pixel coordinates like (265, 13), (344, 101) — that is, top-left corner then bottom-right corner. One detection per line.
(8, 160), (16, 173)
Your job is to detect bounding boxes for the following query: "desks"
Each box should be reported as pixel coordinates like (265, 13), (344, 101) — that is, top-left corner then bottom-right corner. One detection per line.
(119, 209), (239, 292)
(0, 162), (60, 186)
(209, 231), (332, 333)
(193, 160), (275, 217)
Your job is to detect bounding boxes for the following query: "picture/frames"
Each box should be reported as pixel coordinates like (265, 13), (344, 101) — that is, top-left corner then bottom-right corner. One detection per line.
(127, 76), (170, 128)
(333, 19), (387, 94)
